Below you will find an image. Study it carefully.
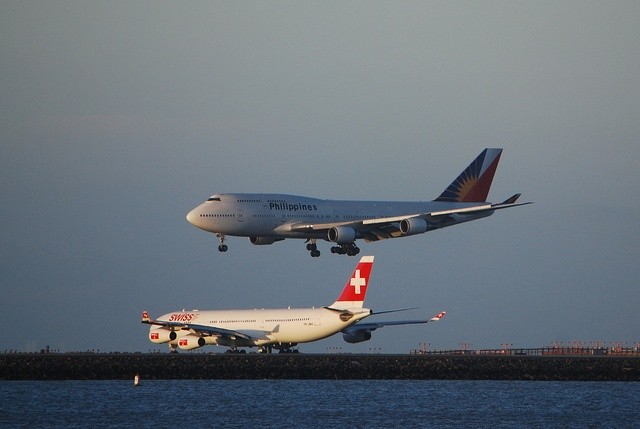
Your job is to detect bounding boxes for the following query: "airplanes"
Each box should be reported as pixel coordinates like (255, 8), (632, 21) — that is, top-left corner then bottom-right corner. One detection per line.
(187, 147), (534, 255)
(142, 255), (446, 352)
